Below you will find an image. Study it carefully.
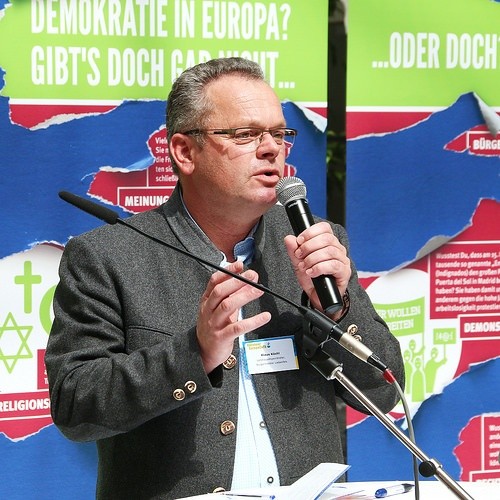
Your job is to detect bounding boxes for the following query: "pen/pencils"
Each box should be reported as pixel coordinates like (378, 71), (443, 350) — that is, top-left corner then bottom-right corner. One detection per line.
(221, 492), (275, 500)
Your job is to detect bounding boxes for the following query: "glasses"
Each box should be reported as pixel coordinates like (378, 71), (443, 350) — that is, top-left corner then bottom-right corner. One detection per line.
(183, 127), (297, 149)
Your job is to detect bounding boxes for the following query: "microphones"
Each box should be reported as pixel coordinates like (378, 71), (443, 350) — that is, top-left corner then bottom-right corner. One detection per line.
(58, 191), (388, 372)
(275, 176), (344, 314)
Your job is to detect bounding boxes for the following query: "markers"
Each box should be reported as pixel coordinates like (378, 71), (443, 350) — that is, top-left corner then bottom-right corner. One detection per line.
(375, 483), (415, 499)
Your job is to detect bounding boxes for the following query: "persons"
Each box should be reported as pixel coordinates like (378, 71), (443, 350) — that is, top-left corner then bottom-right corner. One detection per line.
(45, 57), (406, 500)
